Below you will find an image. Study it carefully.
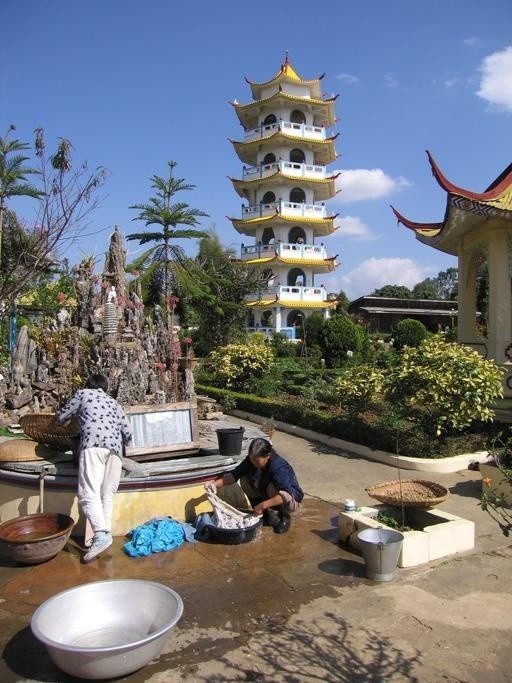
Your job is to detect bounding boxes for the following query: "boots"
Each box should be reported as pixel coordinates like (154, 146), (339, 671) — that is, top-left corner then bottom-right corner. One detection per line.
(262, 506), (290, 534)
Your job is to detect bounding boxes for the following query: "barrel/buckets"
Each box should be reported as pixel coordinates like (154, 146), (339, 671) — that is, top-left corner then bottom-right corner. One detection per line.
(357, 525), (404, 582)
(215, 427), (245, 456)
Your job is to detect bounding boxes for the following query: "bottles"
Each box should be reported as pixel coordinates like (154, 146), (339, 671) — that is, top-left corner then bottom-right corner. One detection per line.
(344, 498), (356, 513)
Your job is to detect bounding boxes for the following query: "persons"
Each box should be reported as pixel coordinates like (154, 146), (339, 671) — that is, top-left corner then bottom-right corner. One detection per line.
(56, 374), (135, 563)
(206, 437), (305, 534)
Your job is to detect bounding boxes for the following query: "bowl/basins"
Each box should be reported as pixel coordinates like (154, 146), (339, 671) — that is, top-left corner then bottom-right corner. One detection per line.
(0, 510), (76, 567)
(28, 576), (185, 678)
(206, 508), (262, 544)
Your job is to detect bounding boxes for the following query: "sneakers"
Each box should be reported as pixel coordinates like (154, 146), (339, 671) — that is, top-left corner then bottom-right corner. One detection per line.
(83, 532), (113, 561)
